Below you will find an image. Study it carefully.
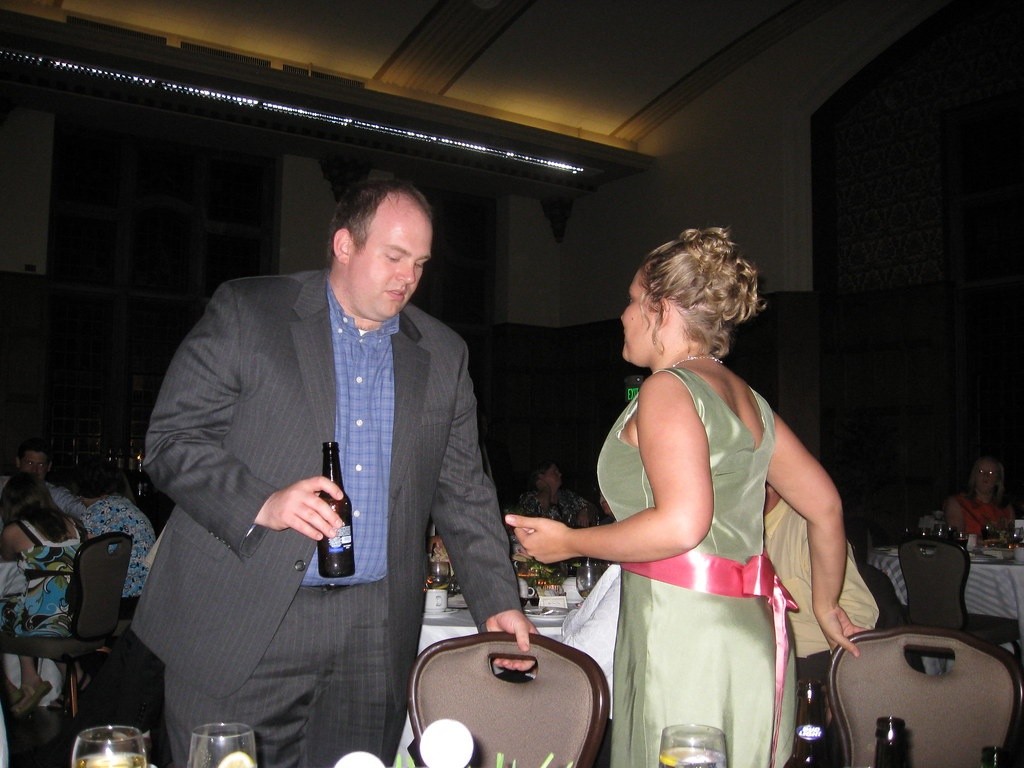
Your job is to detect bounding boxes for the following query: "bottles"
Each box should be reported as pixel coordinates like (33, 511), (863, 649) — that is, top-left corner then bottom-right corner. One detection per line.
(783, 681), (843, 768)
(107, 448), (144, 472)
(872, 717), (907, 768)
(316, 442), (357, 578)
(978, 746), (1005, 768)
(577, 512), (608, 599)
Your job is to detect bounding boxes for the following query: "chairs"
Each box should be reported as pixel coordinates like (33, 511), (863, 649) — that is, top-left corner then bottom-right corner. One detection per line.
(405, 634), (609, 768)
(0, 532), (137, 719)
(856, 559), (906, 627)
(826, 628), (1024, 768)
(897, 540), (1021, 663)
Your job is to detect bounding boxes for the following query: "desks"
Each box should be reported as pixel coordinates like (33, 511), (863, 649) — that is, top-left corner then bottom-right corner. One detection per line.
(862, 529), (1024, 696)
(395, 578), (583, 768)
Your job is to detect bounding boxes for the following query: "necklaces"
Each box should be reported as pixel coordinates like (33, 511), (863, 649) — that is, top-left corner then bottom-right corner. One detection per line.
(672, 356), (723, 368)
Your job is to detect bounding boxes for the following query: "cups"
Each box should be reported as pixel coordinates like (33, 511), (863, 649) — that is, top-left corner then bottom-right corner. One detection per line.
(517, 577), (535, 597)
(188, 723), (258, 768)
(71, 726), (147, 768)
(424, 589), (448, 612)
(576, 566), (602, 600)
(658, 724), (728, 768)
(425, 562), (451, 590)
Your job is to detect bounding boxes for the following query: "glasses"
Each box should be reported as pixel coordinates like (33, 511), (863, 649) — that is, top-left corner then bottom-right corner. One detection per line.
(977, 468), (1000, 475)
(22, 457), (48, 468)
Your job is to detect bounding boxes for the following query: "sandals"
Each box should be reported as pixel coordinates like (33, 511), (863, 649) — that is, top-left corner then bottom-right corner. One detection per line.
(0, 674), (53, 720)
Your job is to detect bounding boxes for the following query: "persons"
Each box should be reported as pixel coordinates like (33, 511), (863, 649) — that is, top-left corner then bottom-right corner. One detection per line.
(503, 228), (866, 768)
(0, 438), (1015, 727)
(130, 180), (541, 768)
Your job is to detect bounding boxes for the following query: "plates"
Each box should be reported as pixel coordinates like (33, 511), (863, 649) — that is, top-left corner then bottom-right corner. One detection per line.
(872, 544), (996, 562)
(422, 610), (457, 618)
(522, 606), (572, 619)
(447, 601), (468, 608)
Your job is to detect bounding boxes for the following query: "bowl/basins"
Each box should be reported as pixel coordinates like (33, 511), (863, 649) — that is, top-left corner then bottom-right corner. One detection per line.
(561, 577), (584, 602)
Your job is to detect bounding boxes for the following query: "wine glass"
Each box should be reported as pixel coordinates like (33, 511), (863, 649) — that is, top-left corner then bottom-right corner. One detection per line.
(908, 520), (1024, 561)
